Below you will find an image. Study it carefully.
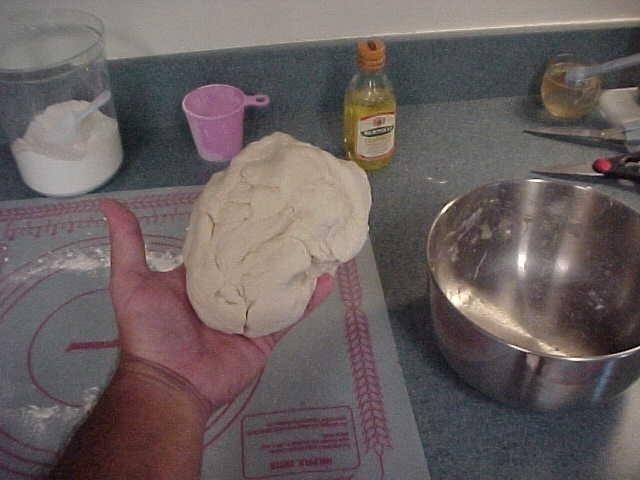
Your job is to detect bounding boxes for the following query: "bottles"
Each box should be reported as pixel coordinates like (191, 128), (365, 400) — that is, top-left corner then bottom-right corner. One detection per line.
(343, 38), (397, 171)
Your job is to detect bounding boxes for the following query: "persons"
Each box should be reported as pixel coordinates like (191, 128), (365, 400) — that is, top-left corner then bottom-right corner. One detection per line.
(47, 195), (337, 480)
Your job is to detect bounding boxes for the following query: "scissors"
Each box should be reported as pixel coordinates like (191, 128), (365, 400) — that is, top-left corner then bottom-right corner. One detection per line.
(522, 118), (639, 154)
(530, 148), (639, 185)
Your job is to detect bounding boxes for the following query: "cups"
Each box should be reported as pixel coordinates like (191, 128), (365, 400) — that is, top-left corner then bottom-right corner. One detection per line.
(542, 53), (602, 124)
(182, 84), (270, 162)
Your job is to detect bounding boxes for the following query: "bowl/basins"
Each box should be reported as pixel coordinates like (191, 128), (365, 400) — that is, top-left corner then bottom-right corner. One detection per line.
(425, 177), (640, 413)
